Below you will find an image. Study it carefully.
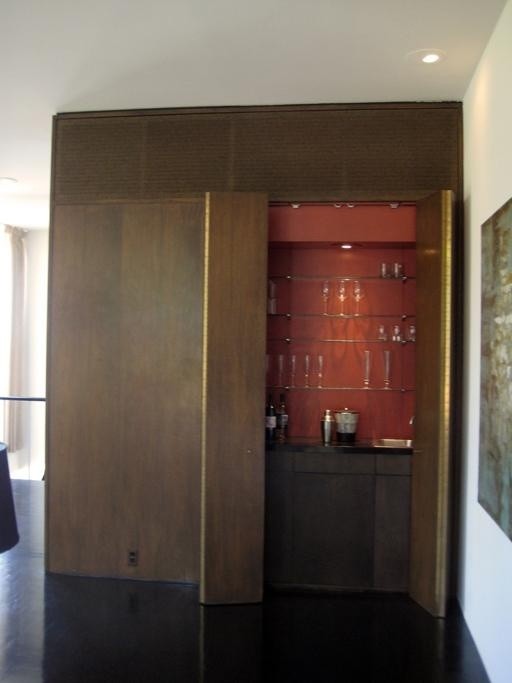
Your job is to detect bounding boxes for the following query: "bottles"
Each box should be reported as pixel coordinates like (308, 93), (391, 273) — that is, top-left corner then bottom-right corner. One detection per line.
(377, 323), (416, 341)
(265, 400), (278, 448)
(279, 399), (288, 444)
(267, 281), (278, 315)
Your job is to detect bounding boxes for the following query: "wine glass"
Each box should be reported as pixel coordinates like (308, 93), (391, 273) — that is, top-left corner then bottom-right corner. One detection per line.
(264, 353), (325, 389)
(360, 350), (392, 390)
(317, 279), (365, 316)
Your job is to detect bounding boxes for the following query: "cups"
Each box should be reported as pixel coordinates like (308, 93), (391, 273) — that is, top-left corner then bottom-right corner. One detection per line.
(319, 409), (336, 445)
(334, 406), (360, 445)
(380, 260), (403, 277)
